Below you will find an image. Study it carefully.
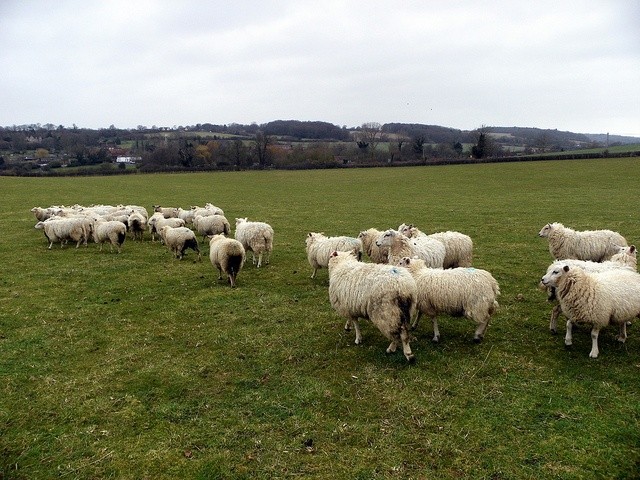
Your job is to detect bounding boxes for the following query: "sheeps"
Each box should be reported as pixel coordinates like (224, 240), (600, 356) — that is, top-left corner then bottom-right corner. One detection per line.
(148, 217), (185, 245)
(147, 211), (165, 245)
(207, 232), (246, 289)
(204, 202), (224, 216)
(151, 204), (178, 218)
(539, 243), (638, 335)
(538, 221), (629, 264)
(357, 227), (389, 264)
(30, 202), (149, 255)
(393, 253), (501, 345)
(376, 228), (447, 269)
(191, 214), (231, 244)
(541, 264), (640, 360)
(304, 229), (364, 280)
(172, 206), (196, 230)
(234, 217), (274, 268)
(427, 230), (473, 270)
(190, 204), (210, 217)
(159, 225), (202, 264)
(326, 248), (418, 365)
(397, 223), (427, 240)
